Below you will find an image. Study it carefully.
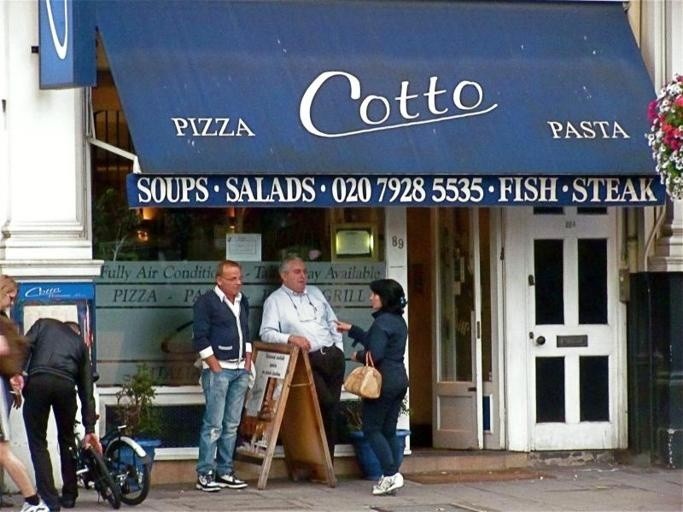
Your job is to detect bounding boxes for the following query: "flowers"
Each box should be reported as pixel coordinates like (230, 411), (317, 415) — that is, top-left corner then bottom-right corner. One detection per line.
(644, 73), (683, 200)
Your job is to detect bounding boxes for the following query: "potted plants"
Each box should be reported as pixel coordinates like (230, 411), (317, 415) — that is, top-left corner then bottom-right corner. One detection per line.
(99, 363), (162, 492)
(339, 376), (416, 481)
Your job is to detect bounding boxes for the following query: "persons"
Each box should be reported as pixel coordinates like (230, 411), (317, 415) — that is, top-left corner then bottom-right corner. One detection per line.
(158, 214), (225, 260)
(0, 273), (30, 507)
(192, 260), (253, 492)
(258, 257), (345, 453)
(244, 212), (321, 262)
(333, 279), (408, 494)
(0, 376), (51, 512)
(19, 318), (102, 511)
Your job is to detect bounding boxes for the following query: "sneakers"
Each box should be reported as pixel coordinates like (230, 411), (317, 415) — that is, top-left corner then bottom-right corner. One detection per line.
(215, 474), (248, 489)
(196, 470), (222, 492)
(372, 472), (404, 495)
(20, 494), (51, 512)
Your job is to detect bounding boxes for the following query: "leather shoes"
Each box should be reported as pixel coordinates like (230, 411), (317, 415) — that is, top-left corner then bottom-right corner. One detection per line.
(49, 500), (75, 512)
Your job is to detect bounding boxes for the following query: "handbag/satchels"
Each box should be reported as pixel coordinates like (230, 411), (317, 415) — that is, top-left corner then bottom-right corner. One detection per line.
(344, 352), (382, 399)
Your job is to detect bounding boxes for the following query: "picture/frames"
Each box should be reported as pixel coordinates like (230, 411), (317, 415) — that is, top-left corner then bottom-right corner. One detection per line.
(330, 222), (380, 264)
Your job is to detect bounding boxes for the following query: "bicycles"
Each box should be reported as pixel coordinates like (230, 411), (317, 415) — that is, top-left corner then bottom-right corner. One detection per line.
(78, 443), (122, 511)
(70, 417), (153, 508)
